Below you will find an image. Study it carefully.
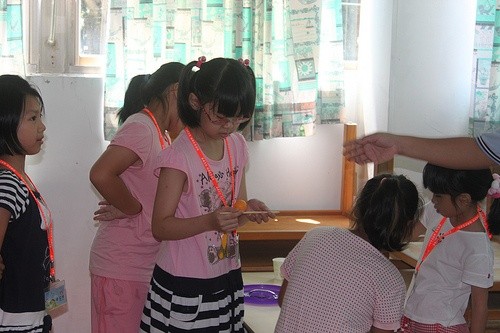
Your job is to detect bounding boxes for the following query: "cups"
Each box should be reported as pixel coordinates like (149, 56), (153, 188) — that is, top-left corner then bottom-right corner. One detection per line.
(272, 258), (285, 280)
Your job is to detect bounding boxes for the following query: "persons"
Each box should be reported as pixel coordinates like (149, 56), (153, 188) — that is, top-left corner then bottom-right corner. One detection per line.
(88, 56), (278, 333)
(275, 173), (424, 333)
(0, 75), (54, 333)
(398, 162), (500, 333)
(343, 126), (500, 170)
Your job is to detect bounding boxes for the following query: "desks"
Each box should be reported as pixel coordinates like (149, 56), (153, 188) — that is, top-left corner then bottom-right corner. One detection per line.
(389, 236), (500, 333)
(241, 272), (284, 333)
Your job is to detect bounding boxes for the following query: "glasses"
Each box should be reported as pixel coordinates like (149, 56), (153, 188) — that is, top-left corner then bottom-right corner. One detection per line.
(200, 105), (250, 125)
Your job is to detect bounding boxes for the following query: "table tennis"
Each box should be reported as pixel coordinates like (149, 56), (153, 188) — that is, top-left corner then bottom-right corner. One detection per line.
(235, 200), (247, 212)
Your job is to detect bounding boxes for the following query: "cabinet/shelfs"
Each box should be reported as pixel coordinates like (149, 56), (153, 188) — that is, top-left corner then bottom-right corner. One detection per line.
(237, 122), (358, 272)
